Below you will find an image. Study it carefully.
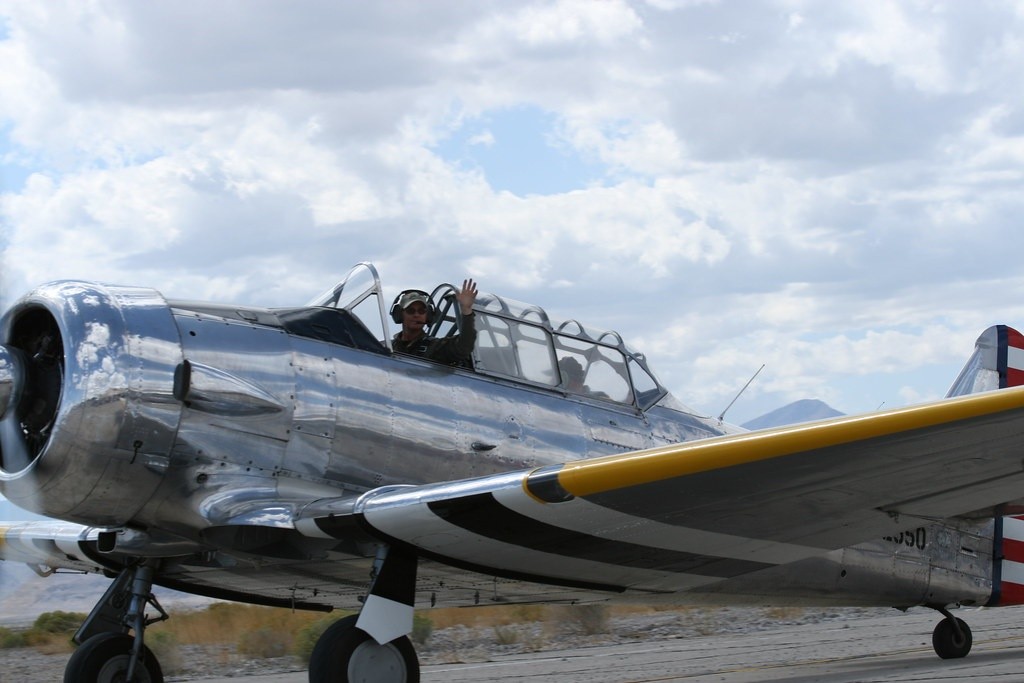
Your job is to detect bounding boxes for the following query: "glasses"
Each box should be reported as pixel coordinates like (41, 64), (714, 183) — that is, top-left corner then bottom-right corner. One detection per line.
(405, 307), (427, 315)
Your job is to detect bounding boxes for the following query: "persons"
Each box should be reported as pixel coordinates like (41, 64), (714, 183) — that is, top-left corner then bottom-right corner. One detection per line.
(541, 356), (608, 398)
(382, 278), (478, 363)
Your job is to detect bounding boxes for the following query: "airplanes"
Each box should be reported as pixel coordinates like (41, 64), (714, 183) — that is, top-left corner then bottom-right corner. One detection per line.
(1, 260), (1024, 683)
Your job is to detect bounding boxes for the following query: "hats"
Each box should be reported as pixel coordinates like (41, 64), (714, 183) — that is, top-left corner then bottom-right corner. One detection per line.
(400, 292), (429, 312)
(543, 358), (582, 377)
